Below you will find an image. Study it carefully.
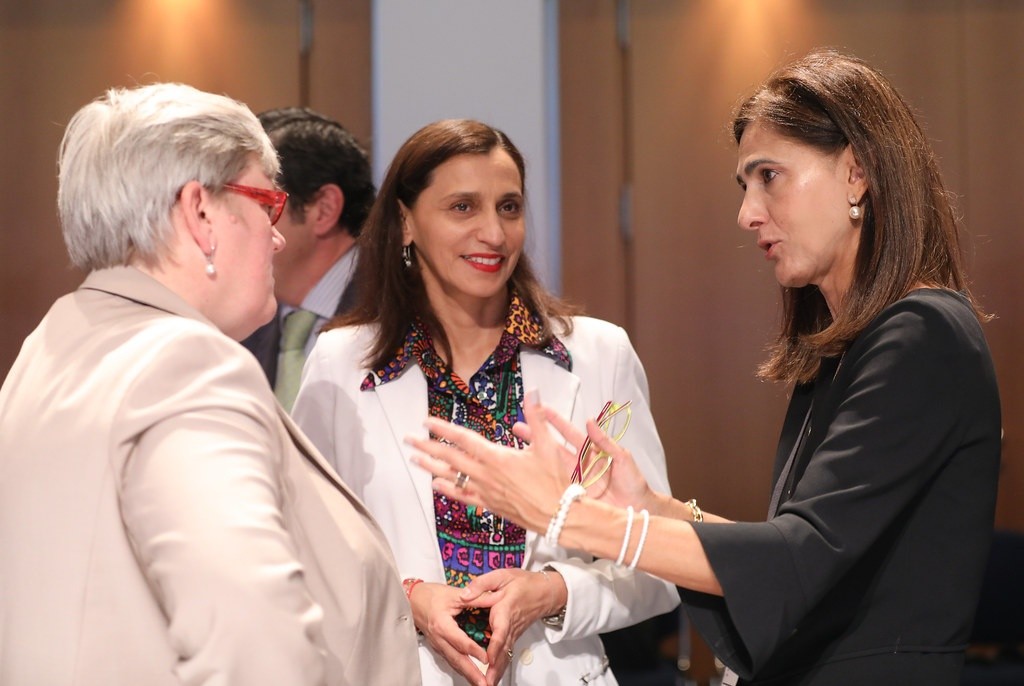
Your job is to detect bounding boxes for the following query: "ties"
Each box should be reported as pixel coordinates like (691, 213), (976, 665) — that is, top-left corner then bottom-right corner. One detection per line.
(273, 309), (320, 415)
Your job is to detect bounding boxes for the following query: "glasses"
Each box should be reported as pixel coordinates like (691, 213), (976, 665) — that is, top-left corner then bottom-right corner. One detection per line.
(176, 182), (289, 226)
(568, 400), (631, 490)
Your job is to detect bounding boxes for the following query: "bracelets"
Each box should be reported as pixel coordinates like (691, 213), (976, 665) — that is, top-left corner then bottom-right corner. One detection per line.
(539, 570), (554, 617)
(614, 505), (649, 571)
(403, 578), (423, 599)
(686, 498), (703, 523)
(544, 485), (586, 545)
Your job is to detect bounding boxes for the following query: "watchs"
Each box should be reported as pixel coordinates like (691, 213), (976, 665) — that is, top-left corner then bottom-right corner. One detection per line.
(541, 603), (566, 626)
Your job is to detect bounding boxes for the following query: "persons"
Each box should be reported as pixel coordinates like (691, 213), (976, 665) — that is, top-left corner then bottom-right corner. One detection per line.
(240, 103), (377, 414)
(0, 82), (422, 686)
(290, 117), (681, 686)
(403, 53), (1002, 686)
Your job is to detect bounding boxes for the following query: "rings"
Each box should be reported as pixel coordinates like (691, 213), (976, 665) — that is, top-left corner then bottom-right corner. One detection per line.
(508, 649), (514, 660)
(455, 472), (469, 488)
